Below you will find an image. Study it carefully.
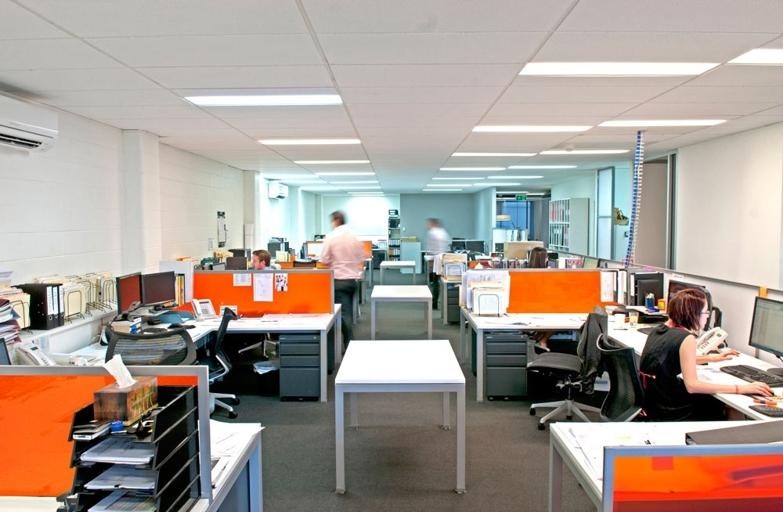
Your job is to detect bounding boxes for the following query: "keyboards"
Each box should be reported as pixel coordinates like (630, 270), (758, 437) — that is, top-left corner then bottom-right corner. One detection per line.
(637, 328), (653, 335)
(140, 328), (166, 336)
(719, 365), (782, 387)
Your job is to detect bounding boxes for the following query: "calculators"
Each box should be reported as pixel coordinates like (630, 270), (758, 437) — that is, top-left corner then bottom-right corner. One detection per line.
(749, 405), (783, 417)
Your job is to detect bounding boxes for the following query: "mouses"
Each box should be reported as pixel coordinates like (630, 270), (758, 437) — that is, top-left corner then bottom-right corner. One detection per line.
(753, 392), (775, 396)
(168, 323), (182, 328)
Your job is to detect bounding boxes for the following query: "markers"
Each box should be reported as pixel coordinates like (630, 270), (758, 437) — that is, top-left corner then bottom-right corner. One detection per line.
(753, 399), (777, 405)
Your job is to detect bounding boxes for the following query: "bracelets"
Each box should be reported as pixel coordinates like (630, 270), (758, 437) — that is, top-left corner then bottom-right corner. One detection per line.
(736, 384), (738, 393)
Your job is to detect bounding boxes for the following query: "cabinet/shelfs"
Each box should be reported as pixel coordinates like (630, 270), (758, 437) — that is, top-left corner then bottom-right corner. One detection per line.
(387, 214), (400, 270)
(549, 197), (589, 256)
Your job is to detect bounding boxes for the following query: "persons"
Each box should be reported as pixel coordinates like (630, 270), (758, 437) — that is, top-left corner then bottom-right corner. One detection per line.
(425, 218), (453, 309)
(527, 247), (555, 352)
(639, 288), (773, 421)
(319, 211), (365, 354)
(252, 250), (274, 271)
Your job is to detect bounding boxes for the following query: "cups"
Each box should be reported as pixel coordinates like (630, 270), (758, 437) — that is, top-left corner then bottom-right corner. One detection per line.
(644, 296), (654, 309)
(629, 311), (639, 326)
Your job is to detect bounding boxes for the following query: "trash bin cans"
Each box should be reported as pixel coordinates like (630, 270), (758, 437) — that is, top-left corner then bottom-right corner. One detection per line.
(254, 361), (279, 396)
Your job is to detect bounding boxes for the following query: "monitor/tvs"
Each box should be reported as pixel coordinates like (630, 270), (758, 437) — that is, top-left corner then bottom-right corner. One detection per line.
(494, 243), (503, 252)
(116, 272), (143, 315)
(305, 241), (322, 259)
(581, 256), (600, 268)
(666, 280), (705, 314)
(748, 296), (783, 377)
(209, 262), (225, 270)
(139, 271), (175, 313)
(0, 338), (11, 365)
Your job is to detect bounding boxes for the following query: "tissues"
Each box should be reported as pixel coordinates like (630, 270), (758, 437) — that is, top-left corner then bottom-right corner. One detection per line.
(94, 354), (158, 427)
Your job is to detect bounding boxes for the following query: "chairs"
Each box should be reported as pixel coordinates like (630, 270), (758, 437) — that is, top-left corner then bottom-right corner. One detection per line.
(450, 238), (485, 253)
(104, 307), (240, 419)
(526, 311), (653, 431)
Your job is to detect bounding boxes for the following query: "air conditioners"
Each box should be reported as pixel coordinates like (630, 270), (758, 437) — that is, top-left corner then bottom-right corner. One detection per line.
(268, 182), (289, 200)
(0, 94), (58, 154)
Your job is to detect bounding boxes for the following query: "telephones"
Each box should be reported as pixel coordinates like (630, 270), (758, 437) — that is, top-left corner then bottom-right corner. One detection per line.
(696, 327), (728, 355)
(191, 299), (216, 319)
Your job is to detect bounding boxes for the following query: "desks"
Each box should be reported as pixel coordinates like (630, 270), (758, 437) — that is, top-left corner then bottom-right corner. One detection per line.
(334, 339), (466, 495)
(440, 251), (783, 512)
(0, 240), (374, 512)
(370, 284), (433, 340)
(379, 260), (416, 284)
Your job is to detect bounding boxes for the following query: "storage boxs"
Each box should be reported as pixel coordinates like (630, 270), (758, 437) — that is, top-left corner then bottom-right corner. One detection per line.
(504, 241), (545, 259)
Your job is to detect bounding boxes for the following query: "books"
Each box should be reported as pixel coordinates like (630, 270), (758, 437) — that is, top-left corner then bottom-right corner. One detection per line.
(0, 298), (21, 343)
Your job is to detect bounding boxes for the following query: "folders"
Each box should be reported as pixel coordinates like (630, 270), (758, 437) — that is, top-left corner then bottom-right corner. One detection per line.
(685, 420), (783, 446)
(10, 283), (65, 330)
